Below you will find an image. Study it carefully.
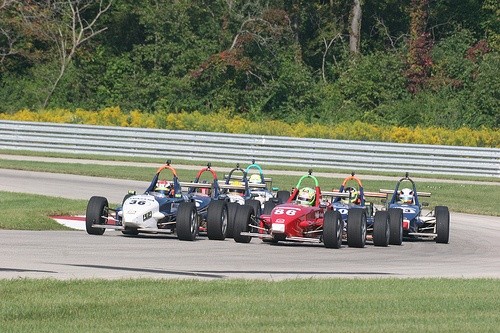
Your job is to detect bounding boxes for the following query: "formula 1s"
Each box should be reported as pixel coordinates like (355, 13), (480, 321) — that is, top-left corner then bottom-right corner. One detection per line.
(85, 164), (450, 249)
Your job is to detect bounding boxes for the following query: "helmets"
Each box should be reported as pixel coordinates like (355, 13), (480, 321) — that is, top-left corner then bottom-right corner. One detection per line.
(228, 180), (243, 196)
(341, 186), (358, 204)
(199, 181), (211, 195)
(295, 187), (316, 207)
(154, 179), (171, 197)
(398, 188), (414, 205)
(248, 174), (262, 191)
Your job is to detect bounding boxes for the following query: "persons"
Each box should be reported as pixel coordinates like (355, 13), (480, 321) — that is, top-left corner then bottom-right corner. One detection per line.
(296, 187), (317, 206)
(248, 174), (262, 191)
(154, 179), (172, 197)
(226, 180), (245, 197)
(341, 186), (359, 204)
(399, 187), (414, 203)
(197, 179), (212, 196)
